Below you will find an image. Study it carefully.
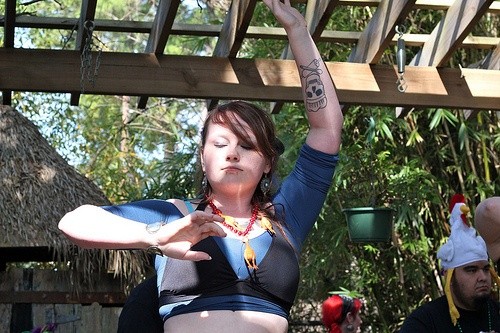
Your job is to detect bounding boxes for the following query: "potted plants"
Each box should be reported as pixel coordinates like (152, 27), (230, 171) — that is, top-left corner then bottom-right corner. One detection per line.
(333, 109), (396, 243)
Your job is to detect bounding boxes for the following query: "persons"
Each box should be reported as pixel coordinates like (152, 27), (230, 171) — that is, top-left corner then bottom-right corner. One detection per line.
(400, 234), (500, 333)
(57, 0), (344, 333)
(322, 292), (363, 333)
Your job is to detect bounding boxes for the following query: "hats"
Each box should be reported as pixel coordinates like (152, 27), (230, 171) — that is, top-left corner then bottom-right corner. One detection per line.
(438, 193), (488, 274)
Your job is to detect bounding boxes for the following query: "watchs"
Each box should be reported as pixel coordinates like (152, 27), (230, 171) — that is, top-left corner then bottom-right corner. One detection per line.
(146, 222), (167, 257)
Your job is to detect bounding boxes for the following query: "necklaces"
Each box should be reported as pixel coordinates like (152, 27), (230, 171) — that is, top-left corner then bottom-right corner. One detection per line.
(447, 293), (495, 333)
(203, 194), (277, 270)
